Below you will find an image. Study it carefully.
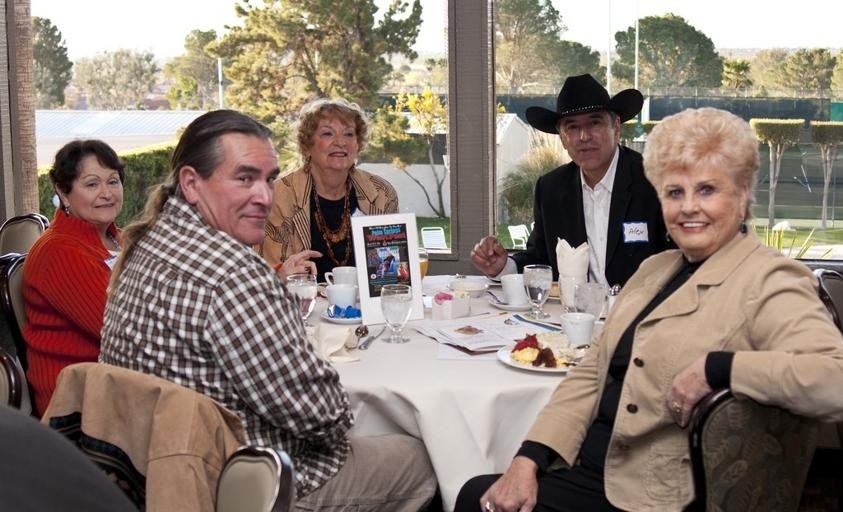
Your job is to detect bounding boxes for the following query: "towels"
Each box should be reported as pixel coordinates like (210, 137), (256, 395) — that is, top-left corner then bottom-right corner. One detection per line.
(305, 325), (348, 358)
(556, 237), (590, 308)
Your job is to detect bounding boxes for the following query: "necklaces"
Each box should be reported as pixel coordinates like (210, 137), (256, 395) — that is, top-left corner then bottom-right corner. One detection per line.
(310, 174), (354, 267)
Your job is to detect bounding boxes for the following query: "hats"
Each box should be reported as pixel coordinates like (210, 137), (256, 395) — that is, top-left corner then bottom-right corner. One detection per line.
(525, 74), (644, 135)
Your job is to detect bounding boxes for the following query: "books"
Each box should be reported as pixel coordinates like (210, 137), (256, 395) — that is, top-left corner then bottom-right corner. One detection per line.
(412, 307), (563, 353)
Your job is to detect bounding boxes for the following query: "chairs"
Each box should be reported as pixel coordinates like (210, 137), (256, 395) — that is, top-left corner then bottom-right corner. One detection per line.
(688, 268), (843, 512)
(0, 213), (293, 512)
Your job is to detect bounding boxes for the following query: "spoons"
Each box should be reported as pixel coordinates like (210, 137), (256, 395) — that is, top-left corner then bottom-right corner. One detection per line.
(350, 325), (369, 351)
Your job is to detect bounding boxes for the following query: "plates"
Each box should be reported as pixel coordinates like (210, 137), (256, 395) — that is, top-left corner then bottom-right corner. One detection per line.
(446, 278), (491, 297)
(487, 295), (532, 312)
(493, 344), (568, 375)
(319, 309), (361, 324)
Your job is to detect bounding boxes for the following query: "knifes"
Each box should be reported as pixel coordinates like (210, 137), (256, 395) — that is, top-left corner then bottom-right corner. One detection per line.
(359, 324), (385, 351)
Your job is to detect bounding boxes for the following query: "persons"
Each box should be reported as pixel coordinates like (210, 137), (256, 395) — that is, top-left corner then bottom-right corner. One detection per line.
(247, 99), (399, 282)
(19, 139), (323, 424)
(468, 89), (680, 294)
(454, 108), (842, 511)
(98, 109), (439, 512)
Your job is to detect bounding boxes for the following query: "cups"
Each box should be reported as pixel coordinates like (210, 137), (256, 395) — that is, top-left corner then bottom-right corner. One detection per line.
(324, 266), (358, 288)
(500, 272), (525, 304)
(325, 283), (358, 313)
(560, 311), (608, 349)
(286, 274), (318, 322)
(557, 274), (588, 312)
(574, 282), (606, 319)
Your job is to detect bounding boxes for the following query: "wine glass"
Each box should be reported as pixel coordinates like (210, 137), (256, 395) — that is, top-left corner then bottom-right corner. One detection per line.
(379, 284), (413, 349)
(417, 248), (427, 297)
(523, 264), (554, 321)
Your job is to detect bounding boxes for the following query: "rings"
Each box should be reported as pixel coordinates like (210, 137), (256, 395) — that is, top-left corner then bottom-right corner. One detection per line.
(671, 399), (682, 412)
(484, 499), (495, 511)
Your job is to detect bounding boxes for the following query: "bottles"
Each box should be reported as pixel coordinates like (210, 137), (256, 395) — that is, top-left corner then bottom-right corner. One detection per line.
(608, 284), (621, 312)
